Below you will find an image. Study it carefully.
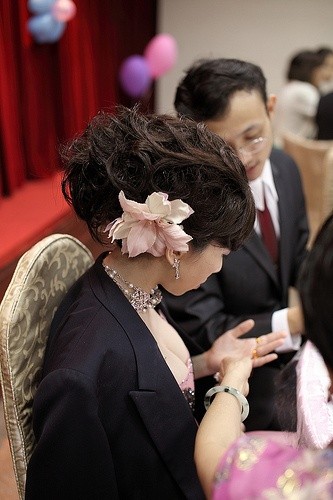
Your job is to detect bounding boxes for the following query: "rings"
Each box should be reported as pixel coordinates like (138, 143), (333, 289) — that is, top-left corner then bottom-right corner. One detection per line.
(252, 350), (257, 358)
(257, 338), (261, 344)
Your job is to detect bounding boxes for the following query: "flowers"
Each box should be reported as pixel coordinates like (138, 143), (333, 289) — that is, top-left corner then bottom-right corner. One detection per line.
(102, 189), (195, 259)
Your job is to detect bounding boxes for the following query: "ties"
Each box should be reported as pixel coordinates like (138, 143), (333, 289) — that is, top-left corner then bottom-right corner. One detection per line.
(255, 181), (278, 262)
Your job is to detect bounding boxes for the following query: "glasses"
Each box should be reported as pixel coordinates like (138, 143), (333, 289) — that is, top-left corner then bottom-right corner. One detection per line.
(234, 114), (270, 156)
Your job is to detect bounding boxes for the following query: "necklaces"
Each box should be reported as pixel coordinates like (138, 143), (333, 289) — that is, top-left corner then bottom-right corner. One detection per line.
(102, 251), (163, 313)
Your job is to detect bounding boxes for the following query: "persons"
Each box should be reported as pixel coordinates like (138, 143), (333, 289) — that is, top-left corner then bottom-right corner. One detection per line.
(269, 46), (333, 148)
(24, 105), (287, 500)
(162, 58), (312, 431)
(194, 211), (333, 500)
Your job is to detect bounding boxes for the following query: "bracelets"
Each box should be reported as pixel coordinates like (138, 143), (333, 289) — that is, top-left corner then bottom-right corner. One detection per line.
(204, 386), (250, 422)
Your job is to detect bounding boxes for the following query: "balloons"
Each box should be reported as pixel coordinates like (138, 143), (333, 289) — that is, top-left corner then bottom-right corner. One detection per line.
(120, 35), (178, 98)
(28, 0), (78, 44)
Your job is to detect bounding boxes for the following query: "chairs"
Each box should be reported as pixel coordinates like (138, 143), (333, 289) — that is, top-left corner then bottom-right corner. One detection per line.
(0, 231), (94, 500)
(284, 134), (332, 246)
